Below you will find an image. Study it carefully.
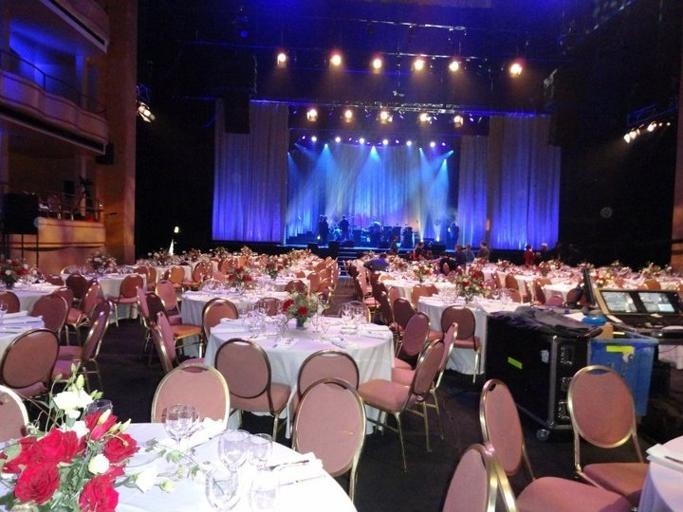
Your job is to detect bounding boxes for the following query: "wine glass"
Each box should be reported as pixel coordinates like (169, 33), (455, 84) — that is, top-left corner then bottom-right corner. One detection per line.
(85, 399), (278, 512)
(237, 302), (365, 340)
(439, 284), (510, 308)
(0, 303), (9, 323)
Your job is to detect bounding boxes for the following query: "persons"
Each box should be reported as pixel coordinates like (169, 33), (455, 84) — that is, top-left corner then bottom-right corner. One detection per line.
(364, 217), (490, 269)
(523, 242), (579, 266)
(318, 215), (350, 245)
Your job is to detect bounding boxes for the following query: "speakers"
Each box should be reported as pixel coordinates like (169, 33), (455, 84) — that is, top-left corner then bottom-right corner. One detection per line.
(95, 143), (114, 164)
(0, 193), (38, 233)
(223, 90), (250, 134)
(549, 99), (577, 147)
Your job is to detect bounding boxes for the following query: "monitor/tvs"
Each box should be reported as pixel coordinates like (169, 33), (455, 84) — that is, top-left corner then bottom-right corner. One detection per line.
(566, 268), (595, 310)
(599, 289), (641, 313)
(636, 292), (676, 314)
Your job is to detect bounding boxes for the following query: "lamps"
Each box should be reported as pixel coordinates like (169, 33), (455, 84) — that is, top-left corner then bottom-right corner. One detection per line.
(622, 117), (673, 144)
(276, 49), (525, 151)
(135, 100), (155, 121)
